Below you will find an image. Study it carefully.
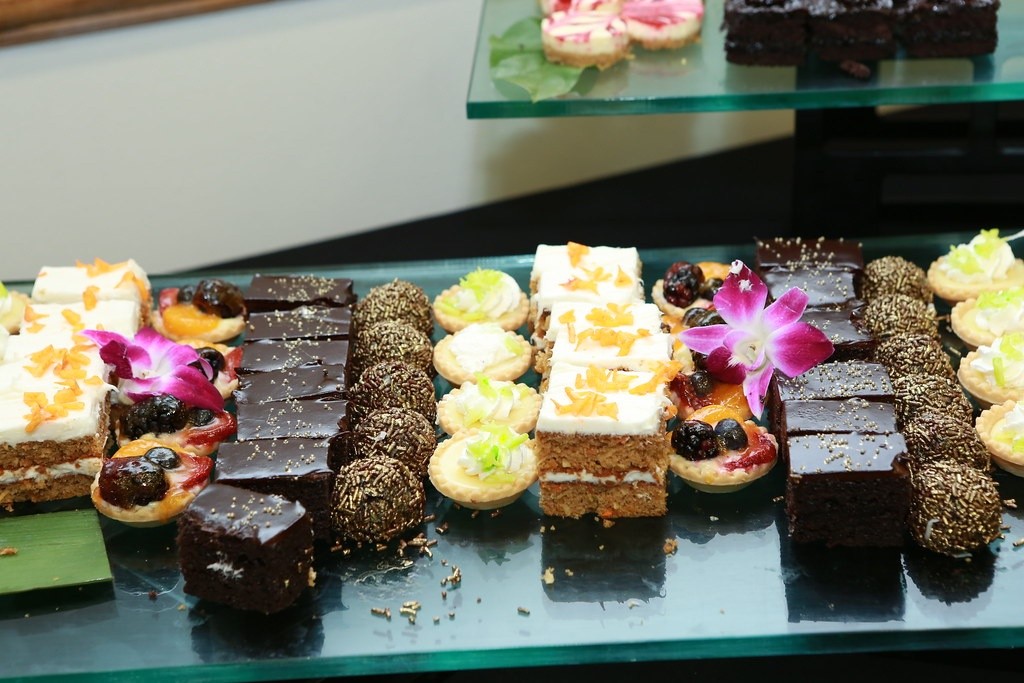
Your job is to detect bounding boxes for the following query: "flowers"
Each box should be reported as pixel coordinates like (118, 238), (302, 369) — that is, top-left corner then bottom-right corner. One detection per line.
(77, 325), (225, 416)
(677, 259), (835, 420)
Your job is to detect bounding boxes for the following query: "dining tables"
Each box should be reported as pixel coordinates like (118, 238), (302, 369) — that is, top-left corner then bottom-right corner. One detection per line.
(0, 234), (1024, 683)
(466, 0), (1024, 239)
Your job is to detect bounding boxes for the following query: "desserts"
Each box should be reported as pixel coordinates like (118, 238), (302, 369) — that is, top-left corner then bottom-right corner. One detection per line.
(0, 227), (1024, 608)
(534, 0), (1001, 71)
